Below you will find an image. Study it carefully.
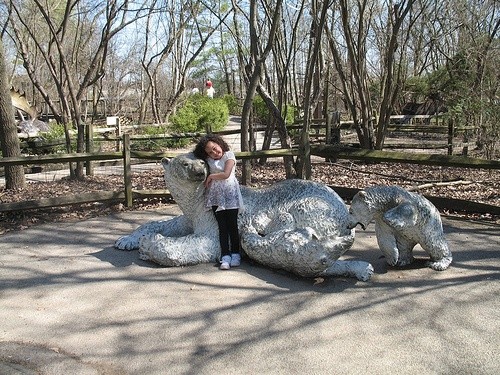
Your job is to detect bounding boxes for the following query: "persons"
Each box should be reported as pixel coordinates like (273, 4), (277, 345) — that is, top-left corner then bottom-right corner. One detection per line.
(194, 134), (245, 270)
(203, 80), (216, 99)
(191, 82), (201, 94)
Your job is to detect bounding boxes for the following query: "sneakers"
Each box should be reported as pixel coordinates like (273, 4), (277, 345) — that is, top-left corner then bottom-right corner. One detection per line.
(231, 253), (241, 266)
(219, 255), (231, 270)
